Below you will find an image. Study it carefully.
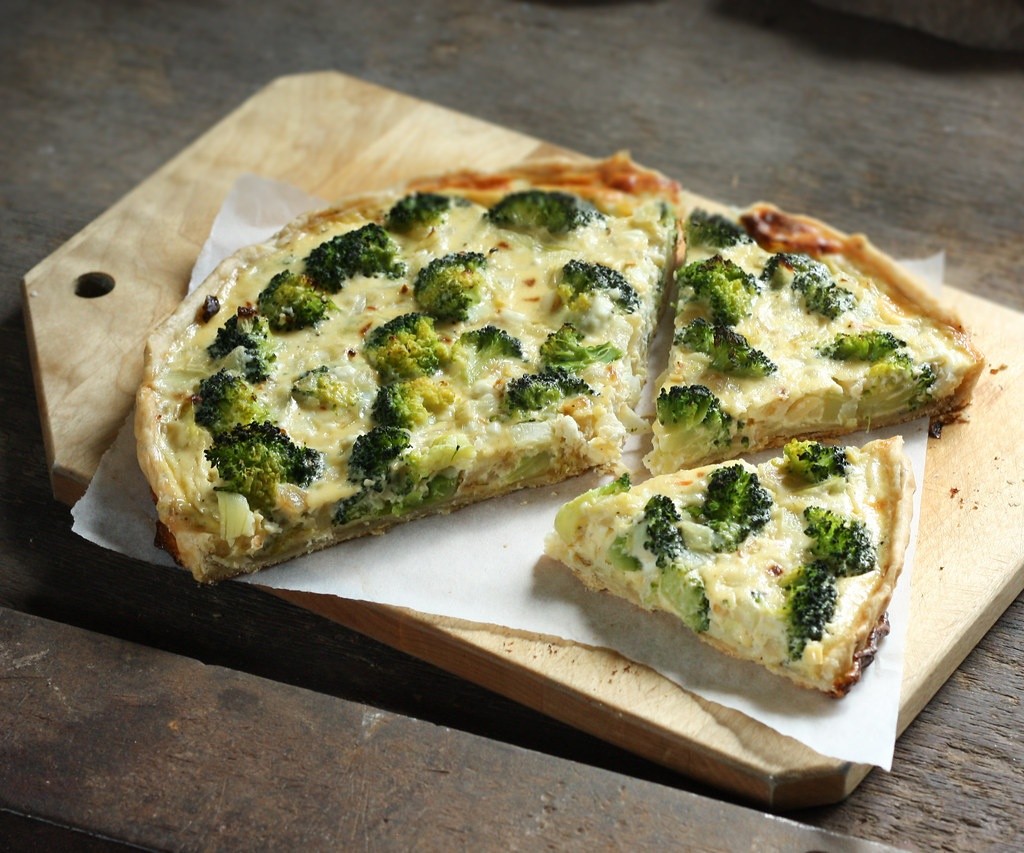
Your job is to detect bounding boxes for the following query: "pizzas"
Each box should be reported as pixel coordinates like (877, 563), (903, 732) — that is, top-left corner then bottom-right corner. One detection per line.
(136, 157), (982, 701)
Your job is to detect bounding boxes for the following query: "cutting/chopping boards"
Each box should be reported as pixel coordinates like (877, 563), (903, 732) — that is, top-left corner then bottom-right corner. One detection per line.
(25, 62), (1024, 804)
